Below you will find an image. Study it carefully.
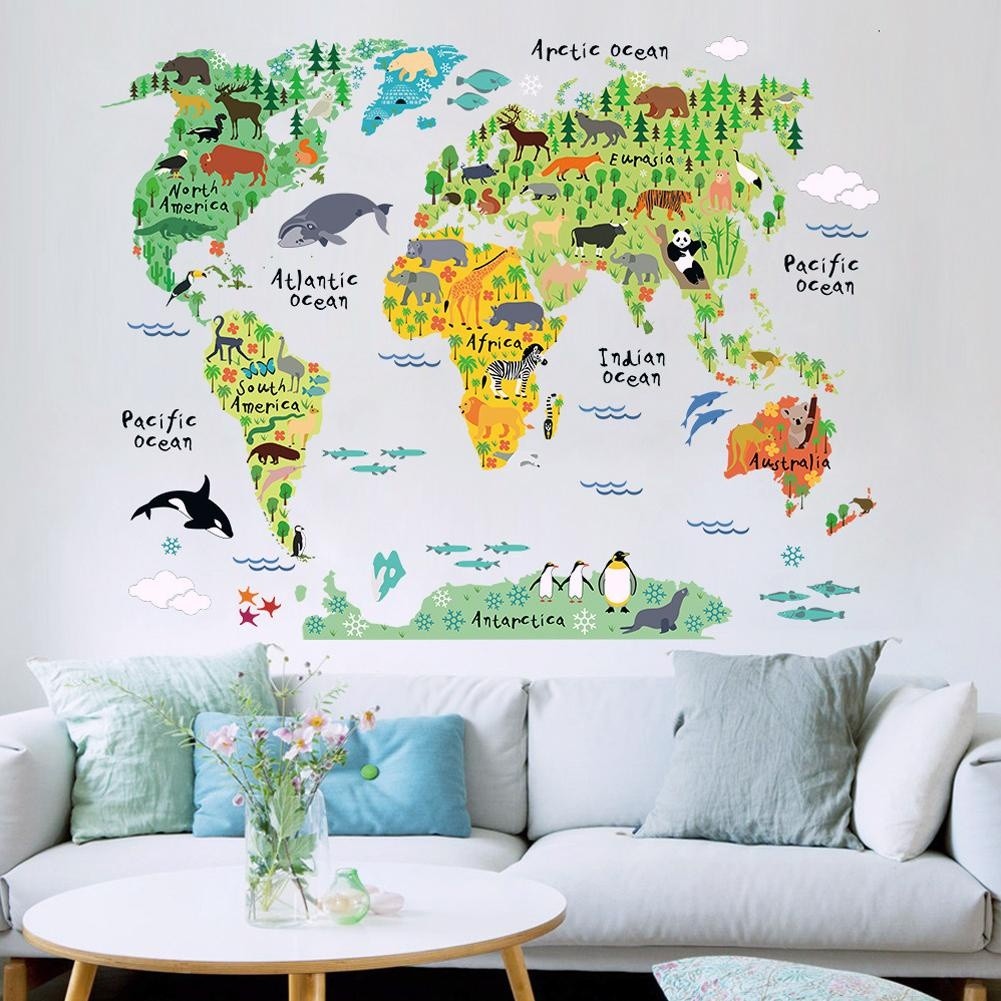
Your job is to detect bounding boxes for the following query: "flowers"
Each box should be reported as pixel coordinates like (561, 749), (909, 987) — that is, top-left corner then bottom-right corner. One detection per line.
(66, 649), (383, 917)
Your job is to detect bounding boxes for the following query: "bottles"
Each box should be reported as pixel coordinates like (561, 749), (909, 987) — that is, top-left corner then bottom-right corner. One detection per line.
(324, 866), (369, 925)
(300, 770), (329, 889)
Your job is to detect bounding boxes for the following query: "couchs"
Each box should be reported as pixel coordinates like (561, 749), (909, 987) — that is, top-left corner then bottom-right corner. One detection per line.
(0, 675), (1001, 1001)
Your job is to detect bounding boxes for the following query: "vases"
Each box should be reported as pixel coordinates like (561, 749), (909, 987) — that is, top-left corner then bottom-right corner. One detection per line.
(243, 786), (326, 929)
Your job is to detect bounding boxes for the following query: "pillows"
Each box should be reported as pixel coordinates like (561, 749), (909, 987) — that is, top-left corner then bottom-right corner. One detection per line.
(28, 640), (977, 865)
(652, 956), (940, 1001)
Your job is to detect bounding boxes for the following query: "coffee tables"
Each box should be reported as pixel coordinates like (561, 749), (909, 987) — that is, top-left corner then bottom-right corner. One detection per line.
(21, 862), (569, 1001)
